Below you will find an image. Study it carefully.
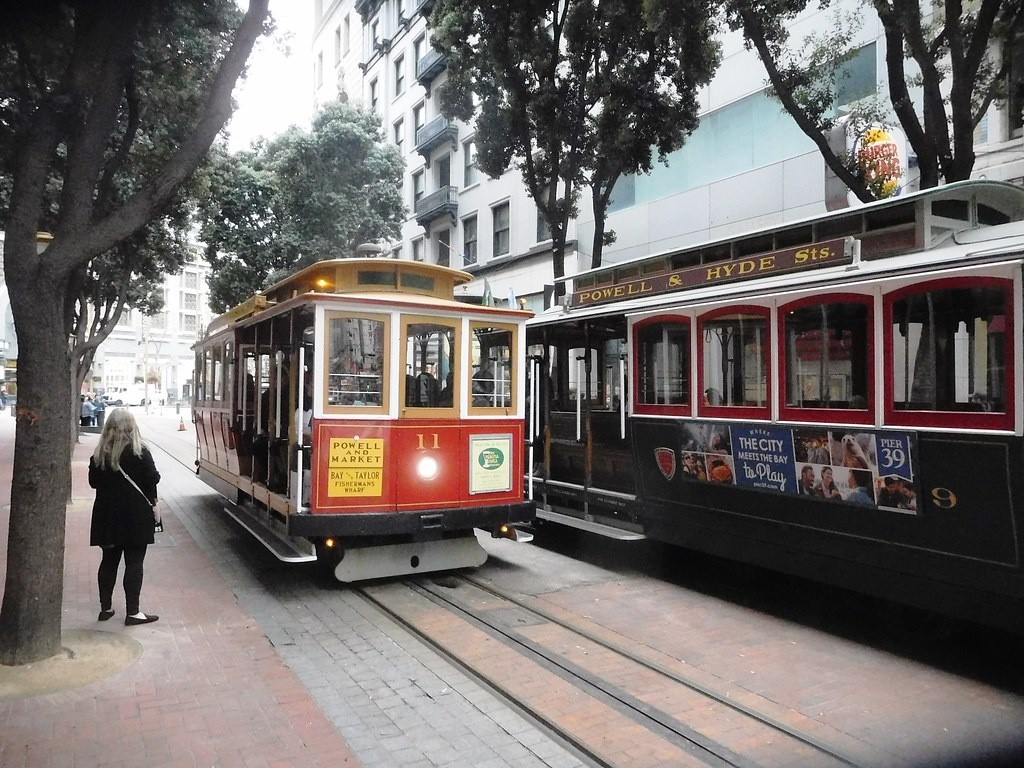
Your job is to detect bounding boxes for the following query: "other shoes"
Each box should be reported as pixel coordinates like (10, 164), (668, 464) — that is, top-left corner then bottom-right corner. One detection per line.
(98, 609), (115, 621)
(125, 613), (159, 625)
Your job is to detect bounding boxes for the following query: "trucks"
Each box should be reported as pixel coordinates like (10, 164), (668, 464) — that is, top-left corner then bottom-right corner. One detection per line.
(102, 384), (155, 407)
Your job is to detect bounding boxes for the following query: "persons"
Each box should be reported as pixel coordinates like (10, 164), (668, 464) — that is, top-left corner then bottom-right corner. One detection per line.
(681, 430), (732, 483)
(88, 408), (160, 626)
(526, 355), (555, 477)
(247, 358), (493, 490)
(798, 436), (916, 511)
(81, 395), (106, 427)
(1, 389), (8, 410)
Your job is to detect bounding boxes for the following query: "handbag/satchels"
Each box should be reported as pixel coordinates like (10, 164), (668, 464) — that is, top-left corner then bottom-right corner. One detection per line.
(152, 506), (162, 532)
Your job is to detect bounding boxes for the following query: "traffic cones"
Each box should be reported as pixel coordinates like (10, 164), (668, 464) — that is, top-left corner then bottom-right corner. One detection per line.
(178, 416), (188, 432)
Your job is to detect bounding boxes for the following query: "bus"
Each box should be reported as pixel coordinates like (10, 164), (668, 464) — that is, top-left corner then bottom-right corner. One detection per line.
(193, 243), (536, 584)
(471, 179), (1024, 645)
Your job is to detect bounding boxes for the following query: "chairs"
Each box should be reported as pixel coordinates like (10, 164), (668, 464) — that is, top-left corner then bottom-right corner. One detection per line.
(707, 384), (868, 408)
